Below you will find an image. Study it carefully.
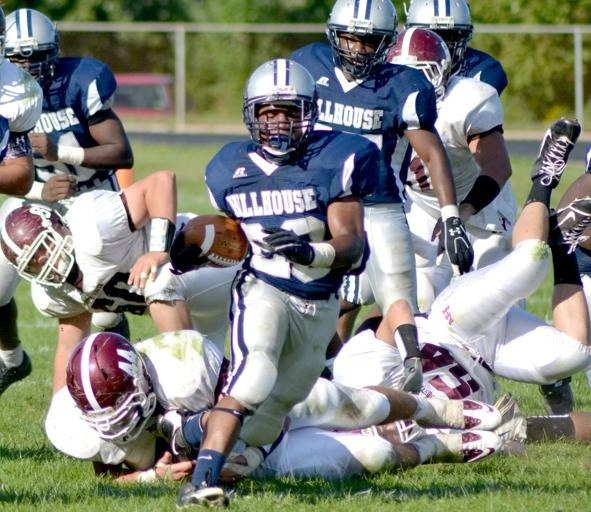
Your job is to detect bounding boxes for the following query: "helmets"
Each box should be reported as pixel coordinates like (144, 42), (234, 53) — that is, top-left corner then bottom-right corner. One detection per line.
(329, 416), (427, 450)
(65, 330), (158, 447)
(0, 5), (9, 67)
(242, 58), (320, 167)
(404, 0), (476, 66)
(0, 203), (74, 291)
(384, 27), (454, 104)
(324, 0), (401, 82)
(4, 7), (61, 87)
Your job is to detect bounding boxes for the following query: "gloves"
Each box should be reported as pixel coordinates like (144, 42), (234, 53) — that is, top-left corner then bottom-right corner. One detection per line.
(436, 215), (474, 278)
(260, 226), (314, 266)
(429, 217), (447, 243)
(167, 230), (210, 275)
(491, 390), (529, 449)
(396, 357), (425, 395)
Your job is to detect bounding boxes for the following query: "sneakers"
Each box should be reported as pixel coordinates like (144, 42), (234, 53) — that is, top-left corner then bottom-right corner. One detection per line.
(174, 481), (230, 511)
(0, 349), (34, 396)
(538, 376), (576, 416)
(414, 428), (504, 464)
(103, 312), (131, 343)
(548, 194), (591, 256)
(415, 396), (504, 432)
(531, 117), (583, 190)
(155, 404), (200, 465)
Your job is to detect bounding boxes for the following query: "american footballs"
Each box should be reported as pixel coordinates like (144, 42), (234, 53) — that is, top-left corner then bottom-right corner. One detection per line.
(179, 213), (248, 270)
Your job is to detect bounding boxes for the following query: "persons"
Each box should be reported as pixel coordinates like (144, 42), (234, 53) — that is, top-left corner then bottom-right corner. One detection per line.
(0, 0), (591, 512)
